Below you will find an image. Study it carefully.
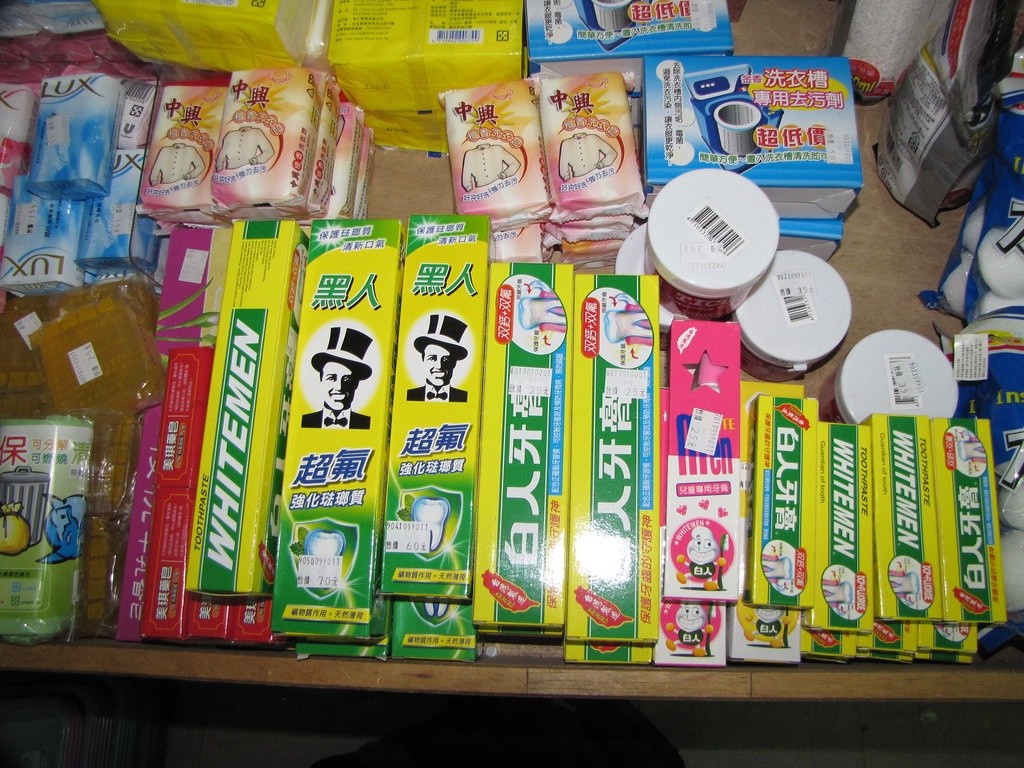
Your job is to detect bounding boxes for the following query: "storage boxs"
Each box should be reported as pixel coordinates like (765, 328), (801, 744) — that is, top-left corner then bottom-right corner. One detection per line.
(115, 0), (1007, 668)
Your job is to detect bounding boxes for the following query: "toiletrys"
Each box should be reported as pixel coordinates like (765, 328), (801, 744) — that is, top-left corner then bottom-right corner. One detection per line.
(187, 214), (1008, 668)
